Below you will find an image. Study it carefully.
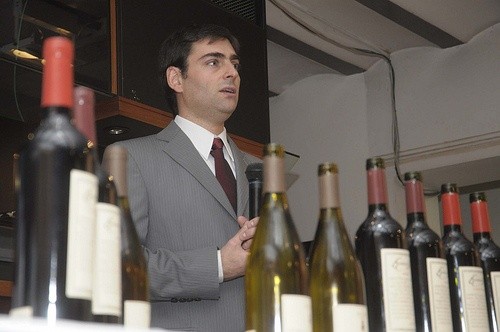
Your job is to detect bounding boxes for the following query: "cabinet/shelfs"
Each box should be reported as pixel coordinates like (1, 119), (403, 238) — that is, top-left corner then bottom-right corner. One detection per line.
(0, 0), (270, 160)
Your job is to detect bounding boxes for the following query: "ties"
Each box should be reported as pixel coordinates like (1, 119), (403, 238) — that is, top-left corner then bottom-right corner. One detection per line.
(210, 137), (237, 218)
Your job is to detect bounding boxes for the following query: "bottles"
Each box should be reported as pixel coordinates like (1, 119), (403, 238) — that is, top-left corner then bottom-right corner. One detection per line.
(8, 32), (97, 322)
(103, 142), (151, 328)
(470, 190), (500, 332)
(72, 83), (124, 324)
(308, 161), (369, 332)
(355, 155), (417, 332)
(244, 142), (312, 332)
(440, 182), (491, 332)
(406, 171), (454, 332)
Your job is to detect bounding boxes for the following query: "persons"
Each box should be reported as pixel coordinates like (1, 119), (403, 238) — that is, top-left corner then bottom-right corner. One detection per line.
(101, 20), (266, 332)
